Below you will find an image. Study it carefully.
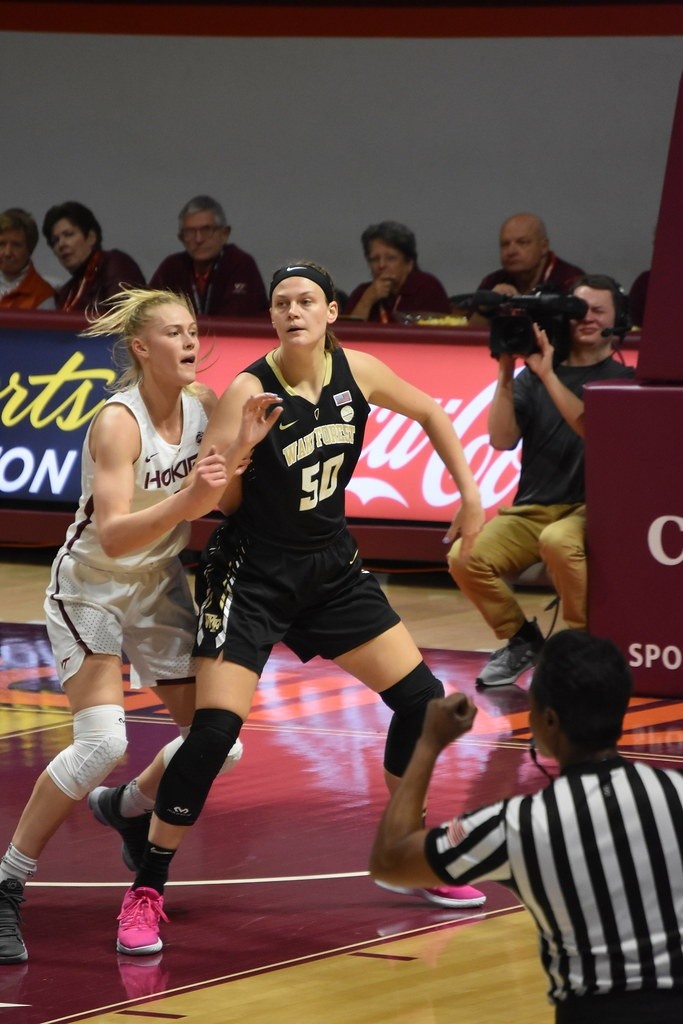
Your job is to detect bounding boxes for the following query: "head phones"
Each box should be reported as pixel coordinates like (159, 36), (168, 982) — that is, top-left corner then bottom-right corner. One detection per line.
(562, 273), (632, 342)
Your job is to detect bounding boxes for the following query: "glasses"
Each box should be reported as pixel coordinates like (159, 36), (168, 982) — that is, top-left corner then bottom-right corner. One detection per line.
(179, 224), (223, 243)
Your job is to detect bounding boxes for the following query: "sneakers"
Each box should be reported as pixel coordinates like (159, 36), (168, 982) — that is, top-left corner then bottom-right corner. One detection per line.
(88, 784), (154, 872)
(116, 884), (170, 953)
(118, 954), (167, 1000)
(0, 878), (28, 962)
(476, 616), (546, 686)
(374, 878), (486, 908)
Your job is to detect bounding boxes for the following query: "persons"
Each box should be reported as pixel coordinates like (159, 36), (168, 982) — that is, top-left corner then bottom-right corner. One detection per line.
(0, 282), (255, 964)
(113, 257), (490, 954)
(370, 630), (683, 1023)
(0, 193), (651, 690)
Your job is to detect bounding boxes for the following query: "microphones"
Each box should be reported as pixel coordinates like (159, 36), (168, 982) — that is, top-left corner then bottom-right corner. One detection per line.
(599, 325), (627, 337)
(474, 289), (507, 305)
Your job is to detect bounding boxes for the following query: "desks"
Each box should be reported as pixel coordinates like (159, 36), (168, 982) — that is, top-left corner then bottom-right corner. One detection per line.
(0, 309), (643, 566)
